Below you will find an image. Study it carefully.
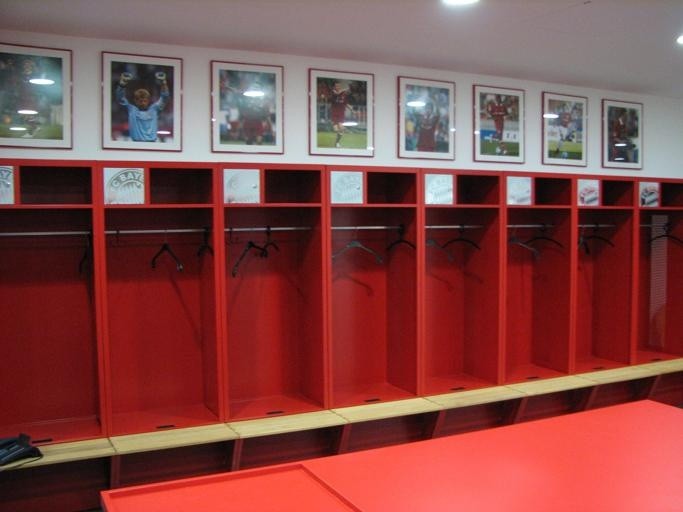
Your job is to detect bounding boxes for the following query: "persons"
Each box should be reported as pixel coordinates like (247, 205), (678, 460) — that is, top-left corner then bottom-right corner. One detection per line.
(487, 93), (509, 155)
(325, 80), (356, 148)
(221, 76), (272, 145)
(413, 98), (441, 152)
(17, 61), (42, 137)
(115, 71), (169, 143)
(554, 104), (574, 158)
(610, 113), (638, 163)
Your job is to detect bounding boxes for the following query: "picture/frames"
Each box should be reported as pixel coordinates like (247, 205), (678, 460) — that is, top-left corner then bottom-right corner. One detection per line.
(210, 60), (285, 154)
(542, 91), (589, 167)
(308, 68), (375, 157)
(0, 41), (73, 149)
(101, 50), (183, 152)
(398, 77), (455, 160)
(601, 97), (644, 170)
(473, 84), (525, 164)
